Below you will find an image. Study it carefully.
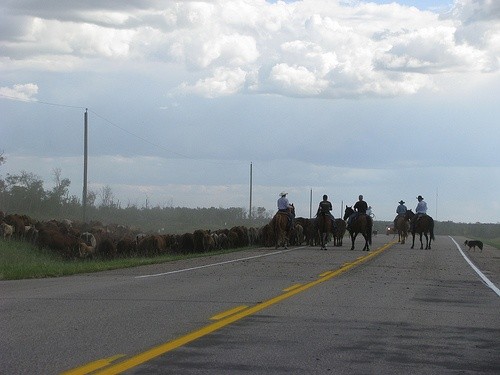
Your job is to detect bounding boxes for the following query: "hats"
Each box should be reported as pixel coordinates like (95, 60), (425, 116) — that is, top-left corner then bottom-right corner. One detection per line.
(399, 200), (405, 204)
(281, 191), (288, 196)
(416, 195), (424, 200)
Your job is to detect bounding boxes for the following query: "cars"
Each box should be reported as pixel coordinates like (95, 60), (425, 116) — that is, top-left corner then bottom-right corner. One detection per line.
(385, 223), (399, 236)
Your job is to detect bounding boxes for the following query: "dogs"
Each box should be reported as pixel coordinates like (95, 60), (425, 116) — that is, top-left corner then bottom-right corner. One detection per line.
(463, 240), (484, 252)
(372, 231), (378, 236)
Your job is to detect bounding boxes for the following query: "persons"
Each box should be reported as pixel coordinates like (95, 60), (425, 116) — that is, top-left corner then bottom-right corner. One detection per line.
(278, 191), (294, 230)
(409, 196), (427, 232)
(318, 194), (336, 234)
(346, 195), (368, 230)
(392, 200), (407, 230)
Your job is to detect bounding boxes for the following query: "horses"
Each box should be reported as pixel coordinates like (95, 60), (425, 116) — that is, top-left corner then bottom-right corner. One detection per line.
(405, 209), (435, 250)
(344, 205), (372, 251)
(1, 209), (346, 261)
(393, 214), (410, 244)
